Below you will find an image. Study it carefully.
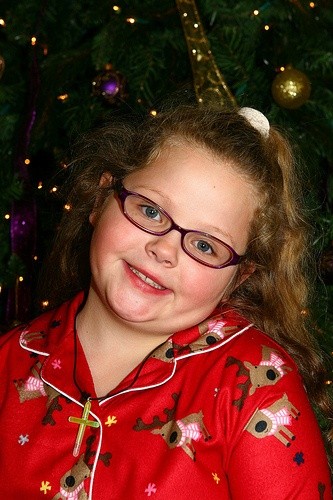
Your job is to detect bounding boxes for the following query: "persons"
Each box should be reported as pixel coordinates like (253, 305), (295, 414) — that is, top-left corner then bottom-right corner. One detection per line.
(0, 100), (333, 500)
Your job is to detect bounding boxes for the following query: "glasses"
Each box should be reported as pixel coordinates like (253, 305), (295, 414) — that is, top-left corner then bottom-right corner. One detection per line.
(115, 180), (247, 269)
